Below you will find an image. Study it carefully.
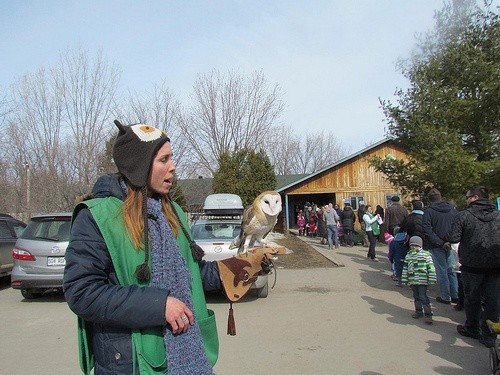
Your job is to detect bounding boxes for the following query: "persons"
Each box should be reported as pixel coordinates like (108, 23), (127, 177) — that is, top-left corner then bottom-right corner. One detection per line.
(446, 185), (500, 339)
(399, 201), (430, 251)
(383, 196), (408, 237)
(323, 198), (389, 250)
(401, 236), (436, 324)
(295, 201), (329, 245)
(63, 120), (286, 375)
(422, 188), (458, 305)
(384, 227), (410, 280)
(362, 205), (380, 261)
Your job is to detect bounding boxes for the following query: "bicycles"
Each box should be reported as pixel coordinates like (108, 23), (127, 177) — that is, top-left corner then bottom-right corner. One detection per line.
(267, 256), (277, 289)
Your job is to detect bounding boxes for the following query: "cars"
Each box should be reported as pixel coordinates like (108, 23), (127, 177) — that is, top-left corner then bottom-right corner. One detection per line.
(0, 215), (29, 279)
(187, 194), (269, 299)
(9, 214), (77, 298)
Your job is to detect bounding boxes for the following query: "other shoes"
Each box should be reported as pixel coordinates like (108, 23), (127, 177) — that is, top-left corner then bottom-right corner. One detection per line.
(367, 256), (379, 262)
(457, 324), (480, 339)
(479, 337), (496, 347)
(412, 310), (423, 318)
(436, 297), (451, 305)
(451, 297), (459, 303)
(424, 314), (432, 323)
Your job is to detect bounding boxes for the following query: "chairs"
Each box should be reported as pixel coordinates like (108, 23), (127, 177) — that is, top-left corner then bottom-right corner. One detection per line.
(233, 226), (242, 237)
(54, 223), (70, 239)
(200, 226), (214, 238)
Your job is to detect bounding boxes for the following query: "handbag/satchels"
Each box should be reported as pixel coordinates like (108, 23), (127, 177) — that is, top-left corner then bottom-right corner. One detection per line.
(353, 220), (361, 232)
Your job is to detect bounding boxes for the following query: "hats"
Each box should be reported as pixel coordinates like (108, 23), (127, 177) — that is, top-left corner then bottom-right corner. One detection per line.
(391, 196), (400, 201)
(409, 236), (423, 248)
(112, 119), (206, 285)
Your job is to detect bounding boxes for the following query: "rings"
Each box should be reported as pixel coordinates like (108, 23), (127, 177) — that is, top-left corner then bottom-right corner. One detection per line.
(182, 316), (189, 323)
